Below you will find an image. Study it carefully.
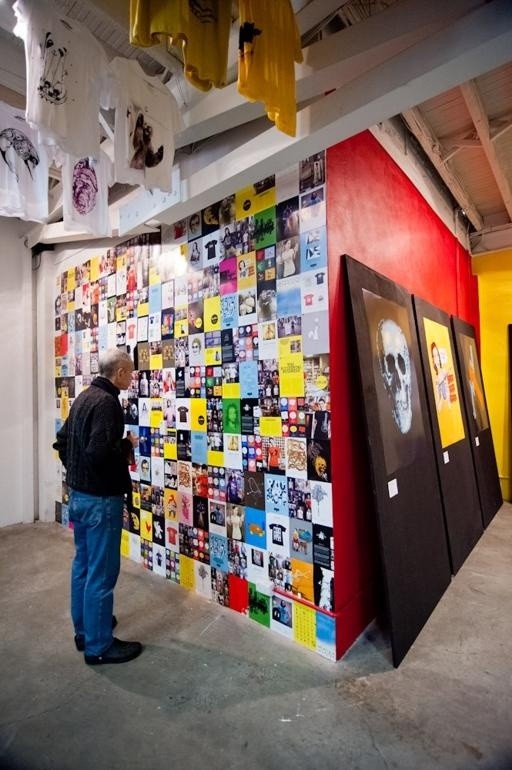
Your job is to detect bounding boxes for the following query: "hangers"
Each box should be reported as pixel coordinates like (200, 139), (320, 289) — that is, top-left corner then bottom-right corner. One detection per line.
(51, 12), (95, 31)
(134, 56), (161, 77)
(0, 100), (30, 121)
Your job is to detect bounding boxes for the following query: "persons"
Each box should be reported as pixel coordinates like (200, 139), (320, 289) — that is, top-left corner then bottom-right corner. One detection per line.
(55, 153), (335, 482)
(465, 341), (486, 433)
(55, 457), (335, 617)
(430, 342), (451, 412)
(52, 349), (143, 665)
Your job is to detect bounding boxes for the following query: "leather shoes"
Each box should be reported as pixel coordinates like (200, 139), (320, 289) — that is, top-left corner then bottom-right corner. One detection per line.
(85, 638), (142, 665)
(74, 614), (117, 651)
